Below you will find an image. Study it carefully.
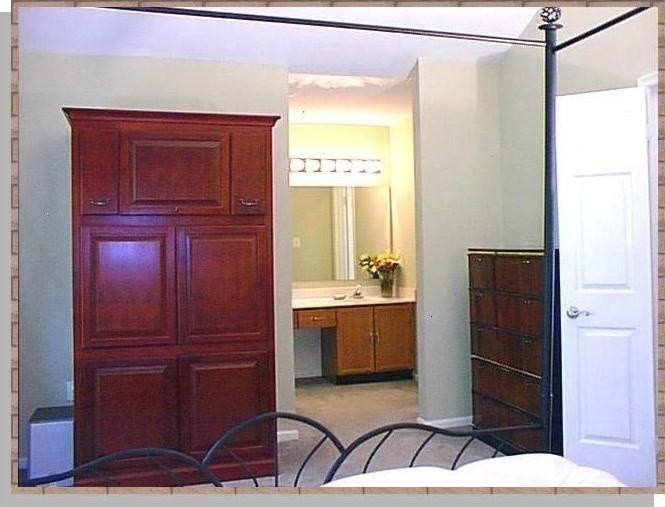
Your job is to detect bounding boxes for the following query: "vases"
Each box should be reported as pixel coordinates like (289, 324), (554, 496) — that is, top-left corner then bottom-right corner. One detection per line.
(381, 280), (393, 298)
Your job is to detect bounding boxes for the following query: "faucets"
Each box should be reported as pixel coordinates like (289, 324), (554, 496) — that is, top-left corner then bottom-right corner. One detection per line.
(346, 284), (362, 300)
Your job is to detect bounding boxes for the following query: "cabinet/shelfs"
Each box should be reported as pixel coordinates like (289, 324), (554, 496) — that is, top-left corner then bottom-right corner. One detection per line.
(467, 249), (551, 456)
(291, 309), (337, 329)
(322, 304), (416, 385)
(61, 105), (282, 487)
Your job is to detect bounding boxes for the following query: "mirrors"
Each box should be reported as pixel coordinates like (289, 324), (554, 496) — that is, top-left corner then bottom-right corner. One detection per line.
(290, 185), (392, 290)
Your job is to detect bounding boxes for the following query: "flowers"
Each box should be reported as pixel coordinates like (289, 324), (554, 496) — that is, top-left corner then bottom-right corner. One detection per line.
(358, 254), (382, 279)
(371, 247), (401, 287)
